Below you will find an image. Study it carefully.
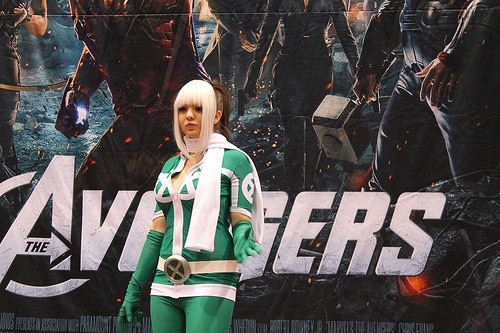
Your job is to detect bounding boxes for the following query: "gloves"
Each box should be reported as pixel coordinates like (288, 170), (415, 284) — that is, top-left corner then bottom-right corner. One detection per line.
(117, 229), (165, 333)
(232, 220), (260, 262)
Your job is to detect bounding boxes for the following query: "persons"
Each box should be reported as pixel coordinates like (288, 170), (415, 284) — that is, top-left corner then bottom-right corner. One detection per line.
(238, 0), (362, 203)
(354, 0), (499, 204)
(115, 78), (264, 333)
(55, 0), (233, 206)
(0, 0), (49, 208)
